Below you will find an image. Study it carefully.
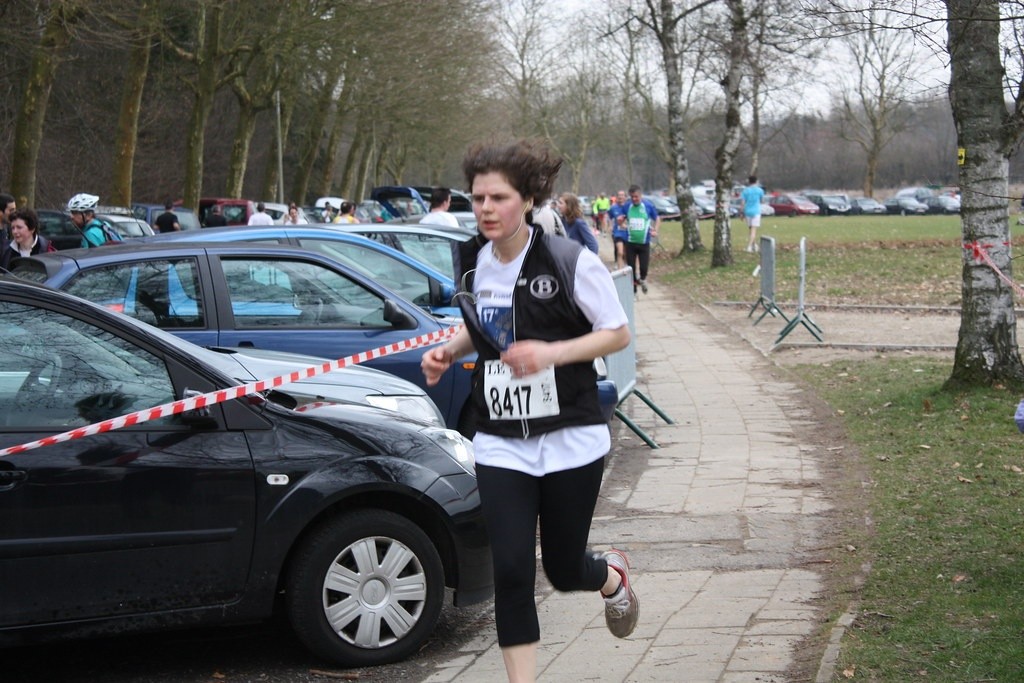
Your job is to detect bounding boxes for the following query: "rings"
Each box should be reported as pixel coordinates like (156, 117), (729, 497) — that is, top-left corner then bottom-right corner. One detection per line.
(521, 363), (525, 378)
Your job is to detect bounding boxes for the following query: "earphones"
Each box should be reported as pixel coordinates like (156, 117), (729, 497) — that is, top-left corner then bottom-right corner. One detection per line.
(526, 200), (530, 207)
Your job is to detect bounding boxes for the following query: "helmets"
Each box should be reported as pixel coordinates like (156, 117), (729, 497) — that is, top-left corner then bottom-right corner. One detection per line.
(68, 192), (100, 212)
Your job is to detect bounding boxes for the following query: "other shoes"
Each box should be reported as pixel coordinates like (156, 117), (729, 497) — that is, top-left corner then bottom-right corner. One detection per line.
(753, 244), (758, 252)
(637, 278), (648, 294)
(747, 246), (752, 253)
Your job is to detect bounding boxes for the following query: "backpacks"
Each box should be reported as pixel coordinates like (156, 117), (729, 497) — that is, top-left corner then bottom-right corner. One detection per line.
(85, 221), (122, 243)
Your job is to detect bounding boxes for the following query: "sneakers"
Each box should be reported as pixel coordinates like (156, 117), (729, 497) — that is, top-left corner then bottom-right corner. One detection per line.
(598, 549), (640, 638)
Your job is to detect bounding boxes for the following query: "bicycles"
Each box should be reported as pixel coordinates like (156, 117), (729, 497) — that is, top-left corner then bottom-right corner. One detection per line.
(599, 211), (608, 237)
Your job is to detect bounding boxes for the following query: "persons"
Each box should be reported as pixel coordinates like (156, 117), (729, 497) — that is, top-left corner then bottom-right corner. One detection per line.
(68, 193), (123, 248)
(419, 188), (460, 228)
(247, 202), (275, 225)
(152, 201), (182, 233)
(283, 201), (309, 224)
(533, 191), (599, 256)
(590, 190), (627, 271)
(617, 185), (660, 300)
(0, 194), (16, 259)
(0, 207), (58, 274)
(741, 176), (765, 252)
(203, 205), (227, 227)
(320, 201), (361, 223)
(420, 139), (640, 683)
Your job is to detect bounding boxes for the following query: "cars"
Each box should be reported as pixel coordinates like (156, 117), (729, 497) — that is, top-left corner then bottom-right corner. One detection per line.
(0, 278), (496, 668)
(30, 184), (476, 337)
(577, 183), (961, 221)
(0, 242), (483, 433)
